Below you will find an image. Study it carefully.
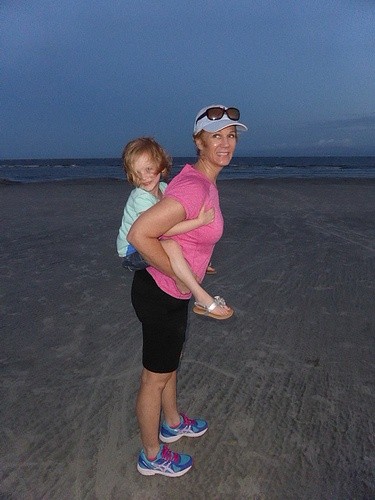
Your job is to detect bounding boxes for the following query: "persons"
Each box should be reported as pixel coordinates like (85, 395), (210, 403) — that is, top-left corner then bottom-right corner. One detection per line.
(126, 105), (249, 477)
(117, 138), (233, 320)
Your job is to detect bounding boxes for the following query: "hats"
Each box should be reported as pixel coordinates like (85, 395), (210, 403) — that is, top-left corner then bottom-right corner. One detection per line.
(193, 104), (248, 136)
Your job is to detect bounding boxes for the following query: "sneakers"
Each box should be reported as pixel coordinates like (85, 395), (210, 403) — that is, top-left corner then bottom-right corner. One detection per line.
(158, 411), (209, 443)
(137, 442), (193, 477)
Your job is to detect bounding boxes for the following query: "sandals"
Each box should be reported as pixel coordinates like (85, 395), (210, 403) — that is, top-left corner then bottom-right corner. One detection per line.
(206, 262), (217, 275)
(192, 295), (234, 320)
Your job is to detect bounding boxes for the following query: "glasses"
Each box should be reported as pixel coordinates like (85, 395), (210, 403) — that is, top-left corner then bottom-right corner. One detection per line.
(196, 106), (241, 126)
